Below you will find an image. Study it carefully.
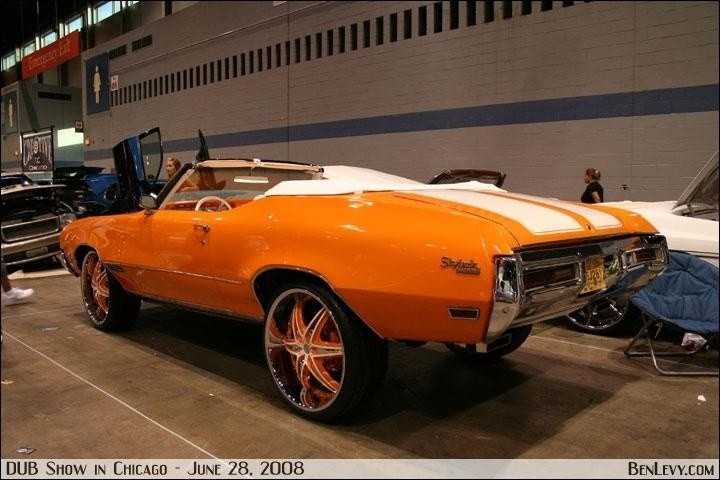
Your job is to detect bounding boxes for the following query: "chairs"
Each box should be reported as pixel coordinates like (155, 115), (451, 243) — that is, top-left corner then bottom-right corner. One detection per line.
(621, 248), (719, 379)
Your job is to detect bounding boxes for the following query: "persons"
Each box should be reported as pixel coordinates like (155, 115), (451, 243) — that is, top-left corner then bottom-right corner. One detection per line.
(24, 135), (53, 172)
(0, 238), (35, 306)
(581, 169), (603, 203)
(166, 156), (199, 192)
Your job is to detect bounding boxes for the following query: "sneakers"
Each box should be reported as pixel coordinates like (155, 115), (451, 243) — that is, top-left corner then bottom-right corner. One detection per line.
(3, 288), (34, 306)
